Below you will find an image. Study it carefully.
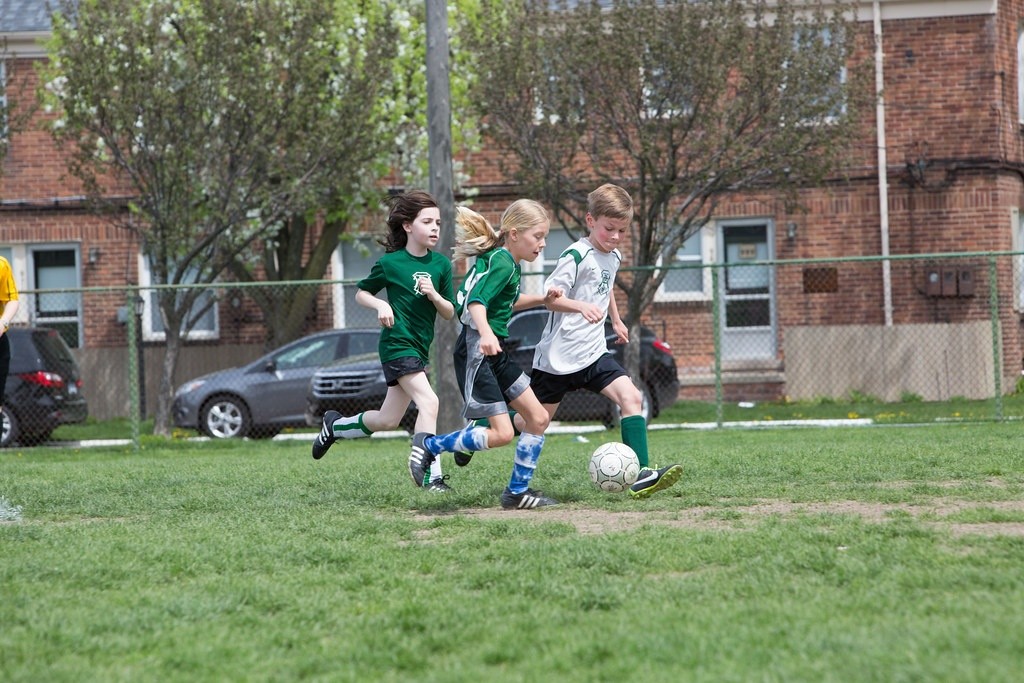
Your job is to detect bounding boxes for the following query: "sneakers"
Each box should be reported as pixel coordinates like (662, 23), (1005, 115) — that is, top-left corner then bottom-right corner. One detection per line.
(409, 432), (435, 488)
(501, 486), (561, 510)
(312, 410), (344, 459)
(424, 474), (455, 496)
(629, 463), (683, 501)
(454, 419), (480, 467)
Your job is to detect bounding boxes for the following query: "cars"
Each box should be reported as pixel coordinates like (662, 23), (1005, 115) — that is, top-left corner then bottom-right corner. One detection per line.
(0, 325), (90, 448)
(301, 304), (682, 432)
(169, 326), (383, 441)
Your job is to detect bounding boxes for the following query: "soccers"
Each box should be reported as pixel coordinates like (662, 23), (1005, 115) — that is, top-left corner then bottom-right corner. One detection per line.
(589, 442), (640, 493)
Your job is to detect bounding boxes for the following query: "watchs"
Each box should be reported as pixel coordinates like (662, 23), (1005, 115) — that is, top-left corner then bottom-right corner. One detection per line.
(1, 318), (9, 328)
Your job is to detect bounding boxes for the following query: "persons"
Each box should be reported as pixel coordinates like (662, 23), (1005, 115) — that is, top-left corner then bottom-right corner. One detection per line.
(455, 183), (683, 499)
(312, 190), (453, 494)
(408, 198), (558, 510)
(0, 256), (20, 439)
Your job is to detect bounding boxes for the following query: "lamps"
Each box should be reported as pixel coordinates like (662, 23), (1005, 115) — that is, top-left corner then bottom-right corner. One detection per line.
(225, 287), (246, 311)
(786, 221), (798, 240)
(87, 246), (100, 267)
(133, 293), (146, 318)
